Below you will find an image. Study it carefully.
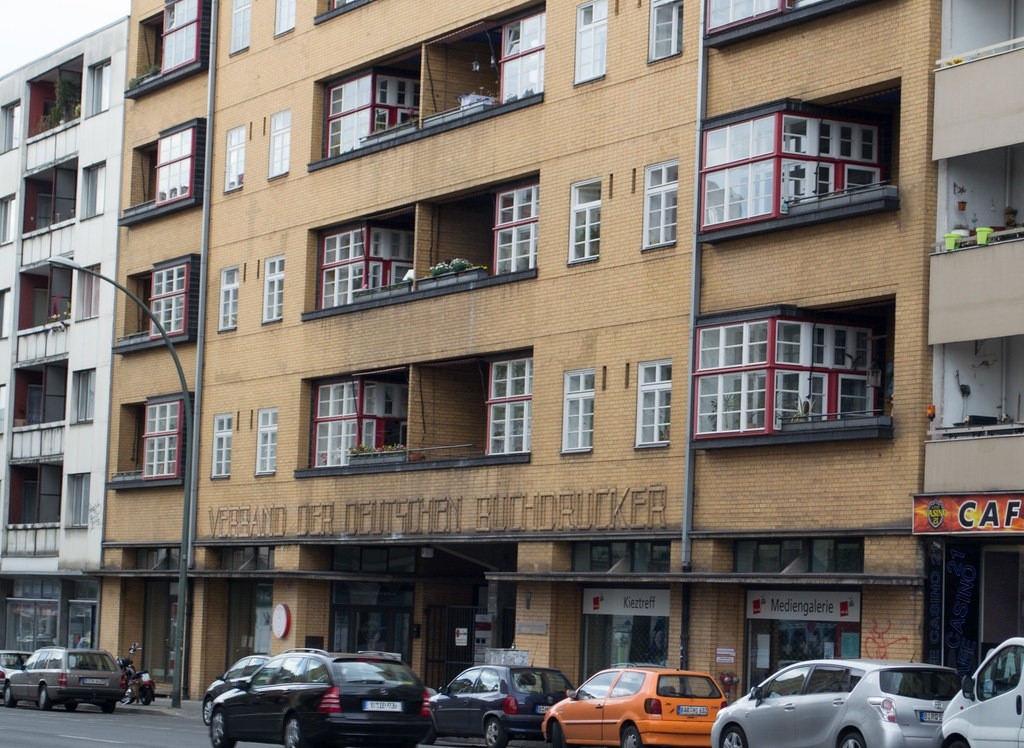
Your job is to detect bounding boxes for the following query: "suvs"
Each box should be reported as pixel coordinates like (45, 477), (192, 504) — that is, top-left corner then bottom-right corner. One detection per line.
(202, 648), (438, 748)
(0, 647), (121, 714)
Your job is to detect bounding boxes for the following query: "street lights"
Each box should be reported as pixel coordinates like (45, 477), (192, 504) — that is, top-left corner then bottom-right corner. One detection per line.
(47, 255), (192, 709)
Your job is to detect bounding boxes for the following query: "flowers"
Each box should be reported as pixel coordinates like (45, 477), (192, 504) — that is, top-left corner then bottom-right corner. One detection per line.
(429, 258), (472, 273)
(349, 443), (405, 453)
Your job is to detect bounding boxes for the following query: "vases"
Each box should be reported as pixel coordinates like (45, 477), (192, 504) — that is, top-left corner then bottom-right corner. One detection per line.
(415, 267), (488, 291)
(346, 453), (405, 464)
(352, 280), (413, 303)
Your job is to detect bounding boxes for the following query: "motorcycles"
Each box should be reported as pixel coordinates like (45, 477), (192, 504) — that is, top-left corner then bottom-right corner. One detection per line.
(116, 642), (157, 706)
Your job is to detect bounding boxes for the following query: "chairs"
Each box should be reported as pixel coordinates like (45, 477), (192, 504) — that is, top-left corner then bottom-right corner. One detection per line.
(659, 686), (678, 697)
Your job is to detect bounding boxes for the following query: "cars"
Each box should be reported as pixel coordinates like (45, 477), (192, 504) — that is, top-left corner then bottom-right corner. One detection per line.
(940, 637), (1024, 748)
(429, 664), (575, 748)
(541, 667), (727, 748)
(711, 659), (962, 748)
(611, 662), (665, 682)
